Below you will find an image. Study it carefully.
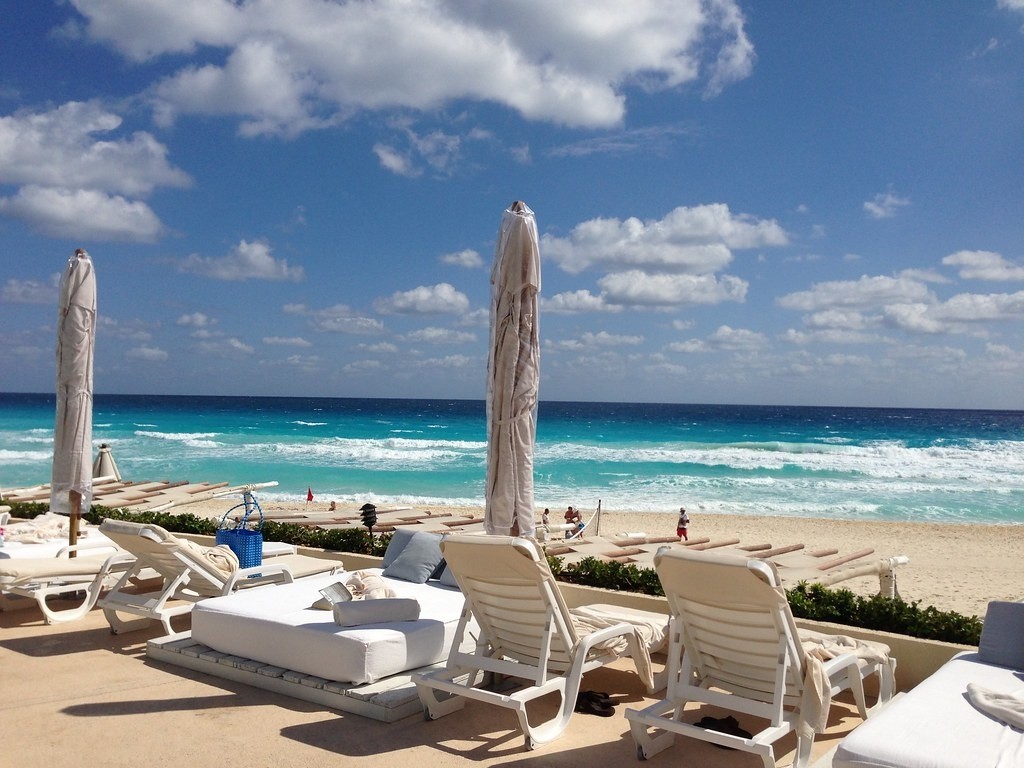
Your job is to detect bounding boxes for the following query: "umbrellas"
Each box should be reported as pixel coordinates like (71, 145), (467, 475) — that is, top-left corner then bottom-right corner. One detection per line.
(48, 248), (95, 559)
(485, 200), (544, 539)
(92, 442), (122, 482)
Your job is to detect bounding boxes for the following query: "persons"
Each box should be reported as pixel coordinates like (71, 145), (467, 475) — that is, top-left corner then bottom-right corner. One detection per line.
(564, 506), (584, 538)
(328, 501), (336, 511)
(676, 507), (690, 541)
(542, 508), (550, 525)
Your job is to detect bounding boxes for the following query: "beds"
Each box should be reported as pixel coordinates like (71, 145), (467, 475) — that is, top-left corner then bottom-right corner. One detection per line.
(189, 568), (490, 686)
(833, 601), (1024, 768)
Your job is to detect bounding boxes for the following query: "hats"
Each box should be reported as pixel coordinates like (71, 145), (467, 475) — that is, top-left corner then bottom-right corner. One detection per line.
(680, 507), (686, 512)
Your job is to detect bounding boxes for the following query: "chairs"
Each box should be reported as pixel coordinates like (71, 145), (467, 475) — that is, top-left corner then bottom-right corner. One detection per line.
(624, 546), (897, 767)
(411, 533), (672, 752)
(0, 504), (164, 624)
(98, 518), (345, 636)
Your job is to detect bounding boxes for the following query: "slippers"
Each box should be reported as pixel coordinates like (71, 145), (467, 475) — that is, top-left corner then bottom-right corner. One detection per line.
(572, 699), (615, 717)
(576, 690), (620, 706)
(693, 715), (753, 750)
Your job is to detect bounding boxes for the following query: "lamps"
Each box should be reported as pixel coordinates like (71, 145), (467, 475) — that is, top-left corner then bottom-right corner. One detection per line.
(359, 503), (378, 527)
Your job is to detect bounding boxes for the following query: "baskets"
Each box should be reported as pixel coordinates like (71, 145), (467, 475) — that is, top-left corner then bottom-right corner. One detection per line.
(215, 492), (264, 578)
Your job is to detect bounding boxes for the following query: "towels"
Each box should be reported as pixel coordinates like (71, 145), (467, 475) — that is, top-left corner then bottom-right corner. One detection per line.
(330, 598), (421, 627)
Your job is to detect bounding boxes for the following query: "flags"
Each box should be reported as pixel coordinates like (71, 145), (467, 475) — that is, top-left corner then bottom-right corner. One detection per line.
(306, 489), (314, 501)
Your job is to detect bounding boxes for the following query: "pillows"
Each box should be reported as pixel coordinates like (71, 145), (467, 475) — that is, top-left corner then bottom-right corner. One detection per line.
(381, 528), (416, 568)
(382, 531), (450, 584)
(441, 564), (458, 586)
